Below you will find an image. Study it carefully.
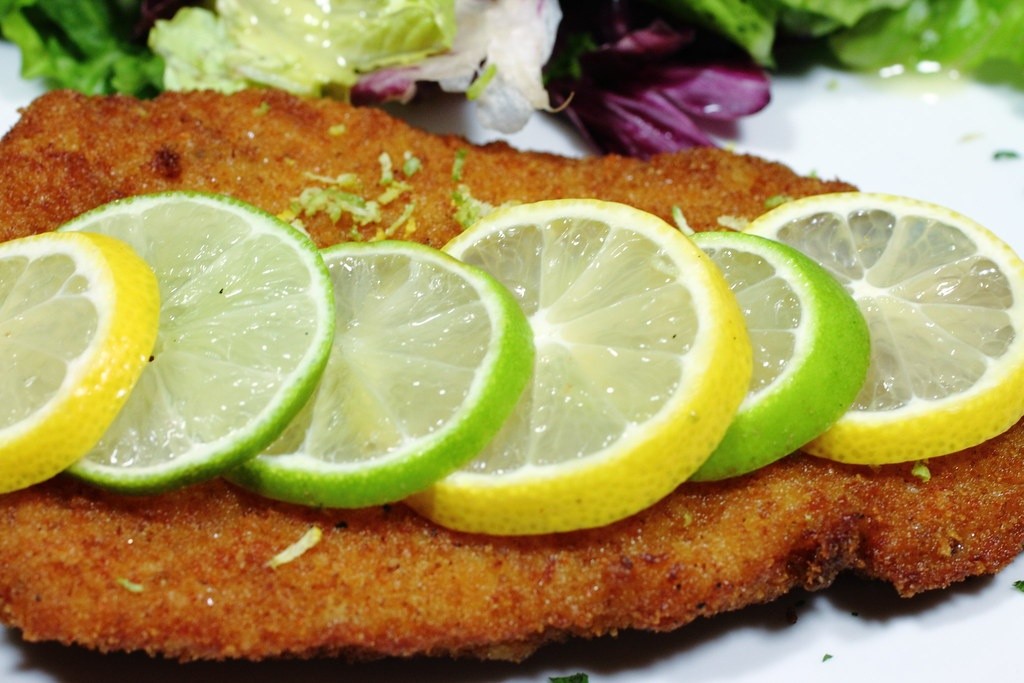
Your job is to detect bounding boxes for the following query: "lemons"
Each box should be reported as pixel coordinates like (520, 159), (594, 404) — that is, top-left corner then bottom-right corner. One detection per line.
(0, 191), (1024, 536)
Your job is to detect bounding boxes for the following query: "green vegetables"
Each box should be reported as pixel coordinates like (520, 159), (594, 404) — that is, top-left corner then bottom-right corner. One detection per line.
(1, 0), (1024, 155)
